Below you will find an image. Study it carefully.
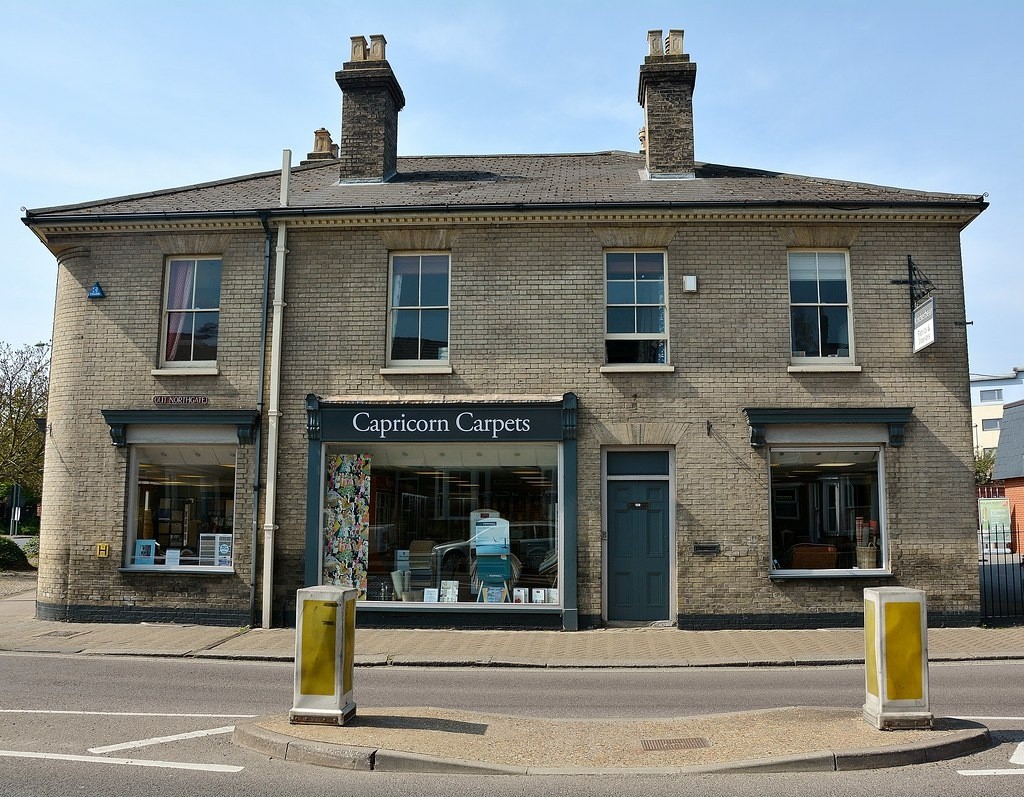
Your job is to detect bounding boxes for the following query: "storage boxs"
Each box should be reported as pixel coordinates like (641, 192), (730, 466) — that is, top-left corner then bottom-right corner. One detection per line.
(856, 542), (877, 568)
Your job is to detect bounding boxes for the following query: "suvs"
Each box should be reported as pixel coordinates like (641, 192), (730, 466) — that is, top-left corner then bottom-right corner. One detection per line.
(431, 521), (558, 574)
